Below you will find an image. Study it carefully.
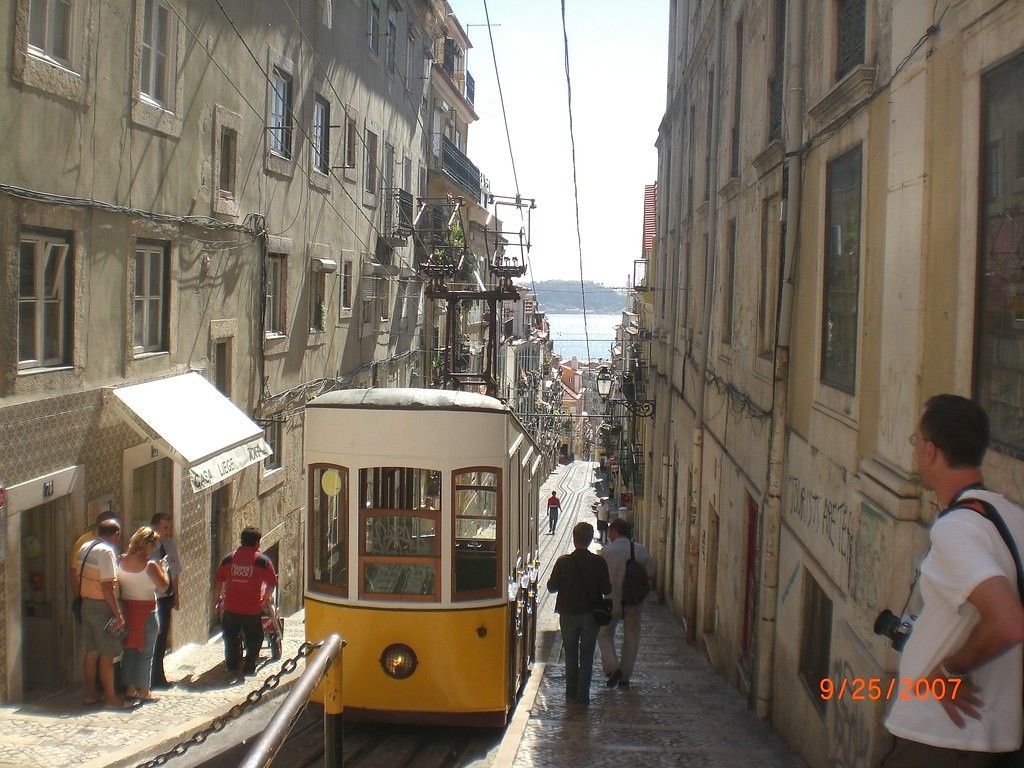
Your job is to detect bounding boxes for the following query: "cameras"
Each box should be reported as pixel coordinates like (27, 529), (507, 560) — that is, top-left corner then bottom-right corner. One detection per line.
(874, 609), (919, 651)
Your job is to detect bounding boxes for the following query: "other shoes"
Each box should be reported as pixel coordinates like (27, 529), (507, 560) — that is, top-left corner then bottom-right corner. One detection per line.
(607, 670), (622, 688)
(597, 539), (602, 541)
(164, 681), (174, 689)
(619, 679), (629, 685)
(227, 666), (239, 673)
(244, 663), (258, 676)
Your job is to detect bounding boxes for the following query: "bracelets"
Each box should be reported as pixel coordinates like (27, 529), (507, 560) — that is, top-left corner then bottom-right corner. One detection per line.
(940, 662), (969, 683)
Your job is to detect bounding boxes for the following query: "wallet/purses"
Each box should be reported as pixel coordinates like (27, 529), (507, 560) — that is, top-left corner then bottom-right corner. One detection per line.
(102, 617), (131, 643)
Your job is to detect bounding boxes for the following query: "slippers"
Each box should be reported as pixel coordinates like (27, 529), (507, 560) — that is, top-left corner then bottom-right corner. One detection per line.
(138, 691), (159, 702)
(105, 700), (133, 709)
(125, 691), (138, 699)
(85, 694), (104, 705)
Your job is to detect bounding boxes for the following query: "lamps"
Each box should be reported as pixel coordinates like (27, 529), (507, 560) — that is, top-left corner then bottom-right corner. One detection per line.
(596, 366), (656, 418)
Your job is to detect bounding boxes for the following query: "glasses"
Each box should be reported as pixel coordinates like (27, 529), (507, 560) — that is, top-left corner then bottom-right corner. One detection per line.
(147, 530), (154, 538)
(908, 433), (939, 448)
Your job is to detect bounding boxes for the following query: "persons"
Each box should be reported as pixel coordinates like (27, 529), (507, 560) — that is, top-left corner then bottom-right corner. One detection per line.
(596, 498), (610, 543)
(547, 491), (562, 535)
(71, 511), (182, 707)
(214, 526), (276, 678)
(546, 523), (614, 707)
(597, 520), (657, 690)
(877, 395), (1024, 768)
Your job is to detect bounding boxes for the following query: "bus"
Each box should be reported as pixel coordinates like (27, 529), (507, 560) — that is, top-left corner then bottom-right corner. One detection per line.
(300, 386), (541, 730)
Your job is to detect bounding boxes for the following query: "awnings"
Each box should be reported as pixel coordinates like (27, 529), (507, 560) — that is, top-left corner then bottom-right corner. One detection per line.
(110, 372), (272, 491)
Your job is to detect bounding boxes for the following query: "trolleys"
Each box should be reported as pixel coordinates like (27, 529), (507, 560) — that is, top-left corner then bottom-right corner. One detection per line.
(216, 597), (283, 659)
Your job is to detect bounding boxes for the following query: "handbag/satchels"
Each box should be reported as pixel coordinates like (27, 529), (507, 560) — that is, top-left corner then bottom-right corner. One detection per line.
(72, 596), (82, 621)
(589, 598), (613, 624)
(623, 538), (650, 605)
